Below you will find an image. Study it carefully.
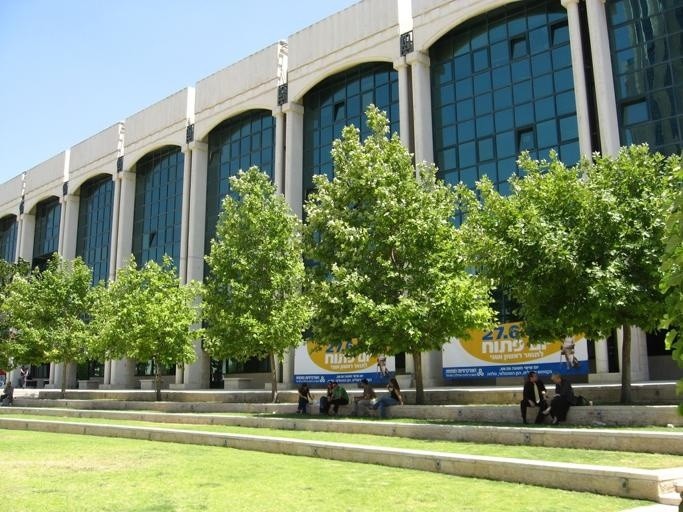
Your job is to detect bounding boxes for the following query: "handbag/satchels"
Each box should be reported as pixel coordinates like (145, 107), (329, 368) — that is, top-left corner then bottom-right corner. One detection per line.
(571, 396), (582, 406)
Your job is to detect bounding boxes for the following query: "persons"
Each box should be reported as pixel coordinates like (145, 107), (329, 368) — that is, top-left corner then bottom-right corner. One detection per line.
(521, 372), (549, 424)
(320, 381), (334, 414)
(296, 382), (313, 415)
(369, 378), (404, 419)
(20, 365), (28, 388)
(540, 373), (574, 425)
(323, 382), (349, 417)
(347, 379), (376, 417)
(0, 382), (14, 406)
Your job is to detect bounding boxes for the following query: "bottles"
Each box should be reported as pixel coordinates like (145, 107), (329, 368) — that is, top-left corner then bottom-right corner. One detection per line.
(667, 424), (672, 428)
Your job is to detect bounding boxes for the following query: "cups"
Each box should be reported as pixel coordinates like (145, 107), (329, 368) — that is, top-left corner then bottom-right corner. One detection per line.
(588, 400), (592, 406)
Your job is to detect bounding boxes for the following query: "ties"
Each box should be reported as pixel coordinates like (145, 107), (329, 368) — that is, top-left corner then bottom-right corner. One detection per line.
(534, 383), (541, 404)
